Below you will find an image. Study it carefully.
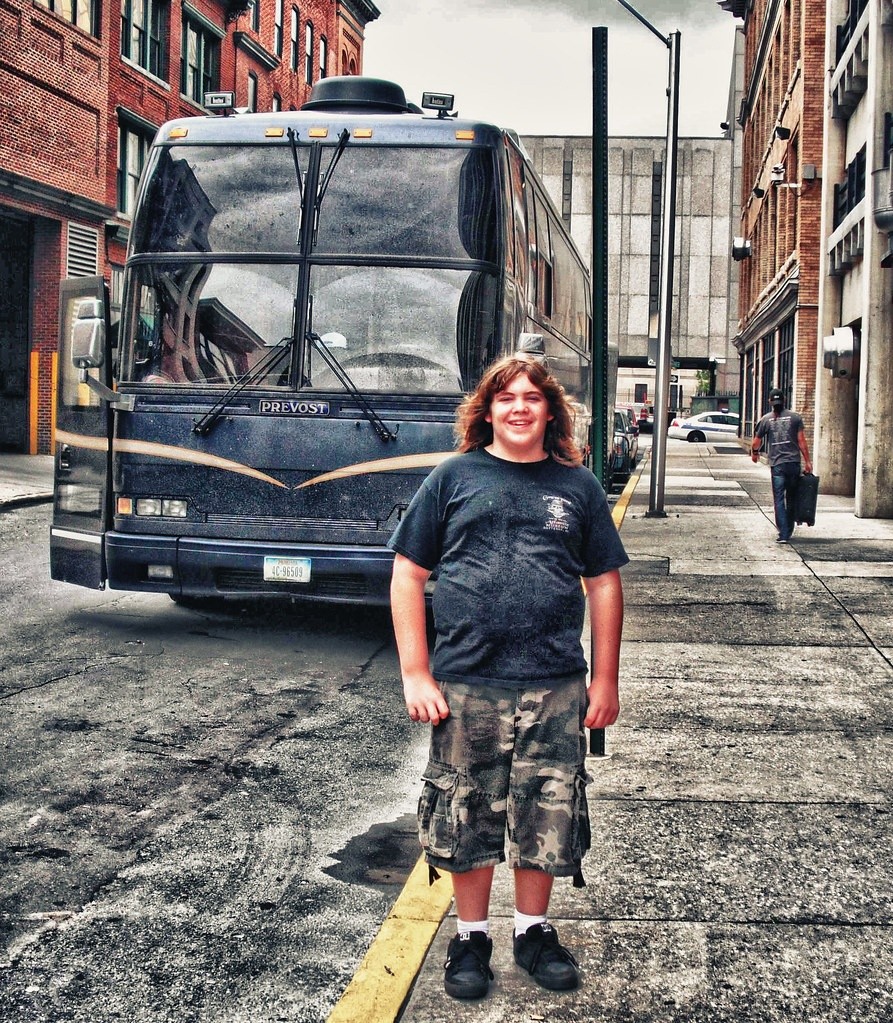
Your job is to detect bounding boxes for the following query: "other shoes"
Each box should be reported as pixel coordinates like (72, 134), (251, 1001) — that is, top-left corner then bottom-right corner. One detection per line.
(776, 533), (791, 543)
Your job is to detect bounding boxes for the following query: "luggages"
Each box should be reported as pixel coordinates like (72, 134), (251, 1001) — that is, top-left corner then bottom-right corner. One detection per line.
(794, 471), (819, 527)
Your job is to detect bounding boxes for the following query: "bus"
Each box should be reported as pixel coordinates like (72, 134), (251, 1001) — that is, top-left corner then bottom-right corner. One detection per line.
(50, 75), (592, 608)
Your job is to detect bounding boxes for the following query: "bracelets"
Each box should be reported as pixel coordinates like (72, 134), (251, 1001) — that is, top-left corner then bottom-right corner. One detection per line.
(752, 449), (758, 455)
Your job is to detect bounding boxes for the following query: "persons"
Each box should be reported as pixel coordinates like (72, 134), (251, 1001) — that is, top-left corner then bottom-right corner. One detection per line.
(752, 388), (813, 543)
(386, 352), (629, 1001)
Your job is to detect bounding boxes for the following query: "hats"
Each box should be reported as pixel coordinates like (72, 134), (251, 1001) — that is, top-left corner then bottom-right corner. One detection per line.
(769, 389), (783, 406)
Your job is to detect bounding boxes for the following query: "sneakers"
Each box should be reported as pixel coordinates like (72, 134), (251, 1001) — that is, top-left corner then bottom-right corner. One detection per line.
(444, 931), (494, 998)
(513, 923), (579, 989)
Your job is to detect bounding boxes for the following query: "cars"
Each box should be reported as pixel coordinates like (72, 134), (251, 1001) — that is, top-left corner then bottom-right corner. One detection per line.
(612, 406), (739, 472)
